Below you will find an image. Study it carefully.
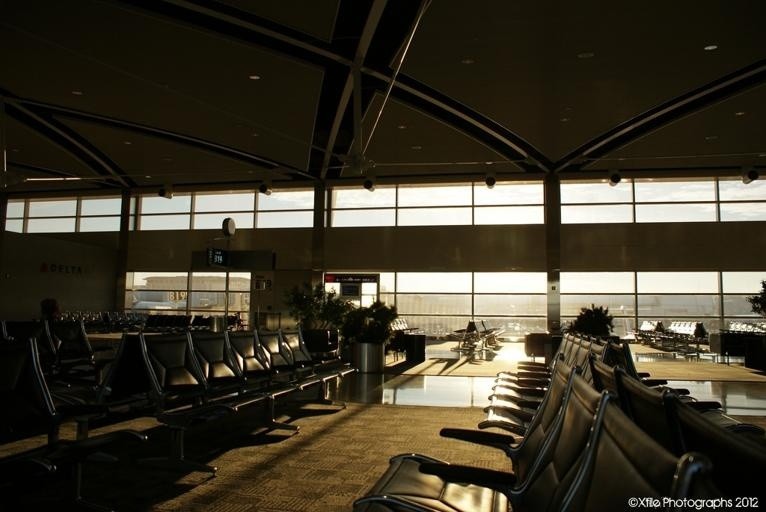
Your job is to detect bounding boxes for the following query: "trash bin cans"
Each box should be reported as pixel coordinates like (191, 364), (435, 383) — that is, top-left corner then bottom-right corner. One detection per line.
(404, 335), (425, 362)
(543, 336), (561, 362)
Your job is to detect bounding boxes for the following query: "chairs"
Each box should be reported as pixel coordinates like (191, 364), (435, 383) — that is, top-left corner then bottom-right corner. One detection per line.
(453, 318), (503, 355)
(390, 317), (419, 361)
(484, 331), (721, 414)
(635, 315), (766, 375)
(356, 414), (766, 511)
(0, 299), (358, 497)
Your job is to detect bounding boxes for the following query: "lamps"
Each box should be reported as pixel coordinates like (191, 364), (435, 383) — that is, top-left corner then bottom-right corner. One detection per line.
(484, 176), (495, 189)
(156, 183), (173, 199)
(363, 178), (375, 192)
(257, 181), (272, 196)
(742, 169), (758, 184)
(608, 171), (621, 186)
